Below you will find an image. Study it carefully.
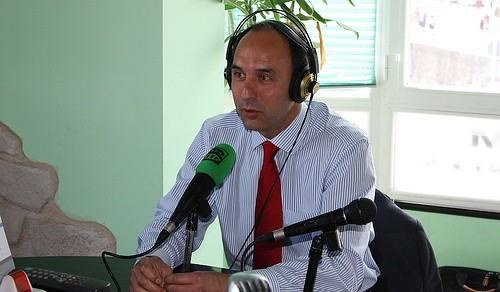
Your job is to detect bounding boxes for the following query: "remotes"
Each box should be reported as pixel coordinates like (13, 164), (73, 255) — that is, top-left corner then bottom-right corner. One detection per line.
(23, 267), (111, 292)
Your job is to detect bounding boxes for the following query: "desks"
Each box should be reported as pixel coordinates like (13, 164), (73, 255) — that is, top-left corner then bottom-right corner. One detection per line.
(11, 255), (243, 292)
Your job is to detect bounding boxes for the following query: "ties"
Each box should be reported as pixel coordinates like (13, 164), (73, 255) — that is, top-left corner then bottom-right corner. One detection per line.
(253, 141), (284, 271)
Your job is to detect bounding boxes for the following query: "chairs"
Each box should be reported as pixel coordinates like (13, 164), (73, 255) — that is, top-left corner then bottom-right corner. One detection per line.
(366, 189), (442, 292)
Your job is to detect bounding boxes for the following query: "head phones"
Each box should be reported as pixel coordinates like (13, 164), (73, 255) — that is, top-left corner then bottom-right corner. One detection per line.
(224, 9), (319, 104)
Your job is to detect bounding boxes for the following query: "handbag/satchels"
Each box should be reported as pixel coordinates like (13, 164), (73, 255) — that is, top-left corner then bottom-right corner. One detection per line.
(438, 266), (500, 292)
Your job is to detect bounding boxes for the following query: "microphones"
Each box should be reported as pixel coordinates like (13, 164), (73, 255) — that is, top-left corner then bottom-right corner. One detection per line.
(259, 197), (377, 242)
(157, 144), (236, 245)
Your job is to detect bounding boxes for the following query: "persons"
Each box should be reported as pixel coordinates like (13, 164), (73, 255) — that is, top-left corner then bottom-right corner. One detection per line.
(129, 9), (382, 292)
